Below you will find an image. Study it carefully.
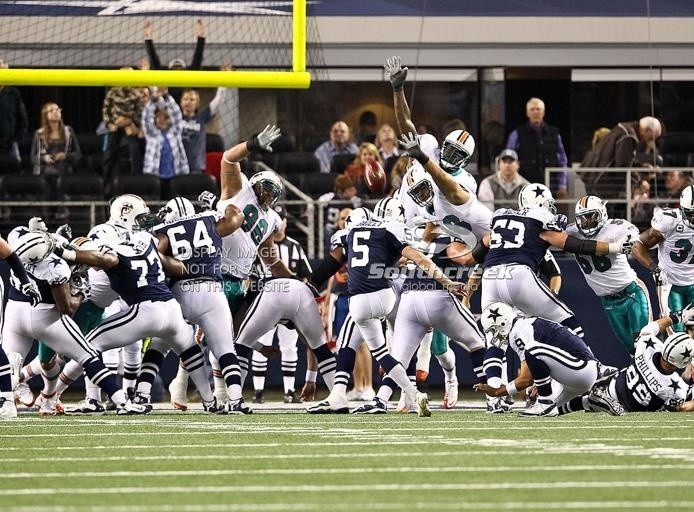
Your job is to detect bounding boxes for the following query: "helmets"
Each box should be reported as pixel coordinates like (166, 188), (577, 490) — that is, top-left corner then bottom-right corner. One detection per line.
(680, 185), (694, 231)
(344, 207), (372, 230)
(110, 194), (154, 231)
(250, 171), (284, 205)
(8, 218), (53, 264)
(439, 131), (478, 170)
(659, 330), (693, 369)
(164, 197), (193, 223)
(573, 195), (607, 236)
(405, 169), (437, 207)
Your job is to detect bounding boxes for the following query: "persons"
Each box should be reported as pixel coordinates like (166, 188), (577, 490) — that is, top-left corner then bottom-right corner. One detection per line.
(0, 18), (694, 419)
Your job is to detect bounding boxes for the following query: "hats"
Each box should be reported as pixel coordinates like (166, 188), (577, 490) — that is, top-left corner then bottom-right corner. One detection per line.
(519, 182), (557, 216)
(498, 149), (517, 162)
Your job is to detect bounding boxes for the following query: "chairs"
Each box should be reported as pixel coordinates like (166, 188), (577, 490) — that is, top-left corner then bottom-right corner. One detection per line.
(0, 88), (693, 232)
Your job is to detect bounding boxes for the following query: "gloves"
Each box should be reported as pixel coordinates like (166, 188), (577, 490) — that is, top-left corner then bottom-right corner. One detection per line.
(610, 234), (634, 256)
(653, 266), (667, 288)
(543, 215), (566, 233)
(398, 131), (429, 167)
(247, 124), (282, 152)
(58, 223), (72, 239)
(199, 191), (219, 211)
(668, 305), (694, 329)
(384, 57), (409, 92)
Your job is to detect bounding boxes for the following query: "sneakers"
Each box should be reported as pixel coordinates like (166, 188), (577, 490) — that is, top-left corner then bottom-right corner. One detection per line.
(284, 375), (459, 417)
(593, 361), (618, 382)
(0, 378), (154, 418)
(168, 377), (264, 417)
(486, 390), (560, 418)
(590, 391), (627, 416)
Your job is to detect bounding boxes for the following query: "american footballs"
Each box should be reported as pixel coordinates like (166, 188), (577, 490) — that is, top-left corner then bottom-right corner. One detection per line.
(363, 156), (387, 198)
